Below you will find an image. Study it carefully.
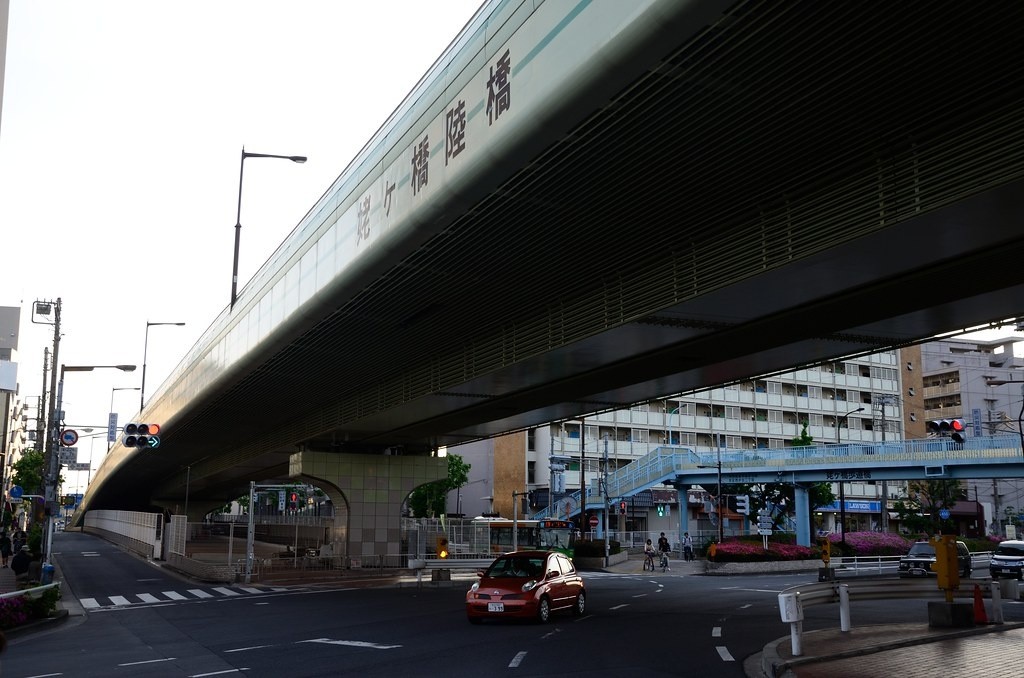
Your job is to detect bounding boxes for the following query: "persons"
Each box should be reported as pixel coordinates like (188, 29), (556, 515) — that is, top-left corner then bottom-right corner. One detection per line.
(0, 531), (11, 568)
(658, 532), (667, 565)
(659, 539), (671, 571)
(13, 527), (28, 554)
(643, 539), (656, 571)
(683, 532), (692, 562)
(11, 545), (35, 591)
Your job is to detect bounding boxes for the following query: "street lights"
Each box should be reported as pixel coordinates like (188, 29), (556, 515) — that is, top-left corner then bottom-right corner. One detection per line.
(106, 388), (141, 450)
(837, 407), (866, 549)
(668, 404), (686, 444)
(231, 147), (307, 304)
(140, 321), (186, 413)
(54, 365), (137, 439)
(697, 461), (723, 542)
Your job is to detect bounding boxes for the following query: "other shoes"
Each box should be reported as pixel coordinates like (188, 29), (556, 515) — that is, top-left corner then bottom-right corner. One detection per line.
(667, 567), (671, 571)
(643, 564), (645, 570)
(2, 564), (8, 569)
(659, 563), (664, 568)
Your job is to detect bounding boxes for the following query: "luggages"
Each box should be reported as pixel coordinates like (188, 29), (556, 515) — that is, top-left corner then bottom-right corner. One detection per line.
(690, 551), (696, 561)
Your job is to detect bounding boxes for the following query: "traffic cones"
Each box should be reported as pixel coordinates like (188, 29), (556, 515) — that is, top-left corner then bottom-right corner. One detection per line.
(974, 583), (993, 625)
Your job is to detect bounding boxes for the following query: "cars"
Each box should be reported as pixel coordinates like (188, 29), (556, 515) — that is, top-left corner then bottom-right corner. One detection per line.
(464, 550), (588, 624)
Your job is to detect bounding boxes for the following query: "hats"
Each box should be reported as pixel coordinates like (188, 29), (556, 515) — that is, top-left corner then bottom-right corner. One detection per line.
(21, 545), (30, 551)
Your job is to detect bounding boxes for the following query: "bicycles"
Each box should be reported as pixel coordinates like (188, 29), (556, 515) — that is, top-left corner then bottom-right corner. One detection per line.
(643, 551), (654, 572)
(659, 551), (669, 572)
(684, 544), (693, 562)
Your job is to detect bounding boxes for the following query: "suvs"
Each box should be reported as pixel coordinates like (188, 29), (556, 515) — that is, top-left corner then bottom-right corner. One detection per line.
(989, 540), (1024, 580)
(896, 541), (972, 578)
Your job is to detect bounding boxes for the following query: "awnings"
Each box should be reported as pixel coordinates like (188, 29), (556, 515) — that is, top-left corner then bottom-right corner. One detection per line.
(888, 511), (924, 520)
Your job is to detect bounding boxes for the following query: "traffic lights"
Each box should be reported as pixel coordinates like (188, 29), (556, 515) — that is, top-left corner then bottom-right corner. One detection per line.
(290, 493), (299, 511)
(928, 420), (967, 443)
(124, 424), (162, 436)
(438, 537), (447, 558)
(619, 501), (626, 514)
(817, 536), (830, 563)
(123, 437), (160, 448)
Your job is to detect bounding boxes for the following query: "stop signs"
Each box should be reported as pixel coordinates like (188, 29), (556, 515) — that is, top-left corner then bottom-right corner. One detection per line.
(589, 517), (599, 527)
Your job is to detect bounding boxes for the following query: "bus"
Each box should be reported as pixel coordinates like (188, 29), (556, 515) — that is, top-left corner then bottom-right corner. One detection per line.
(469, 515), (575, 564)
